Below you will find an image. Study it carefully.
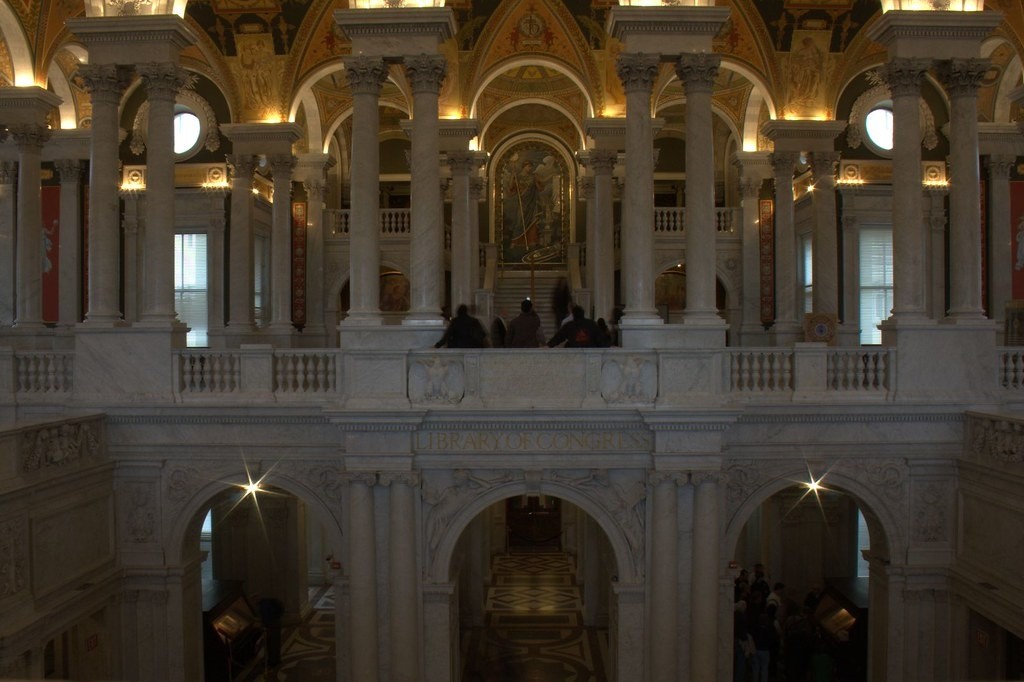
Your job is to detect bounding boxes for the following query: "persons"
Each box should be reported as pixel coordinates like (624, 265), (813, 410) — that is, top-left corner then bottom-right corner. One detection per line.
(733, 563), (822, 682)
(503, 278), (623, 348)
(434, 304), (486, 348)
